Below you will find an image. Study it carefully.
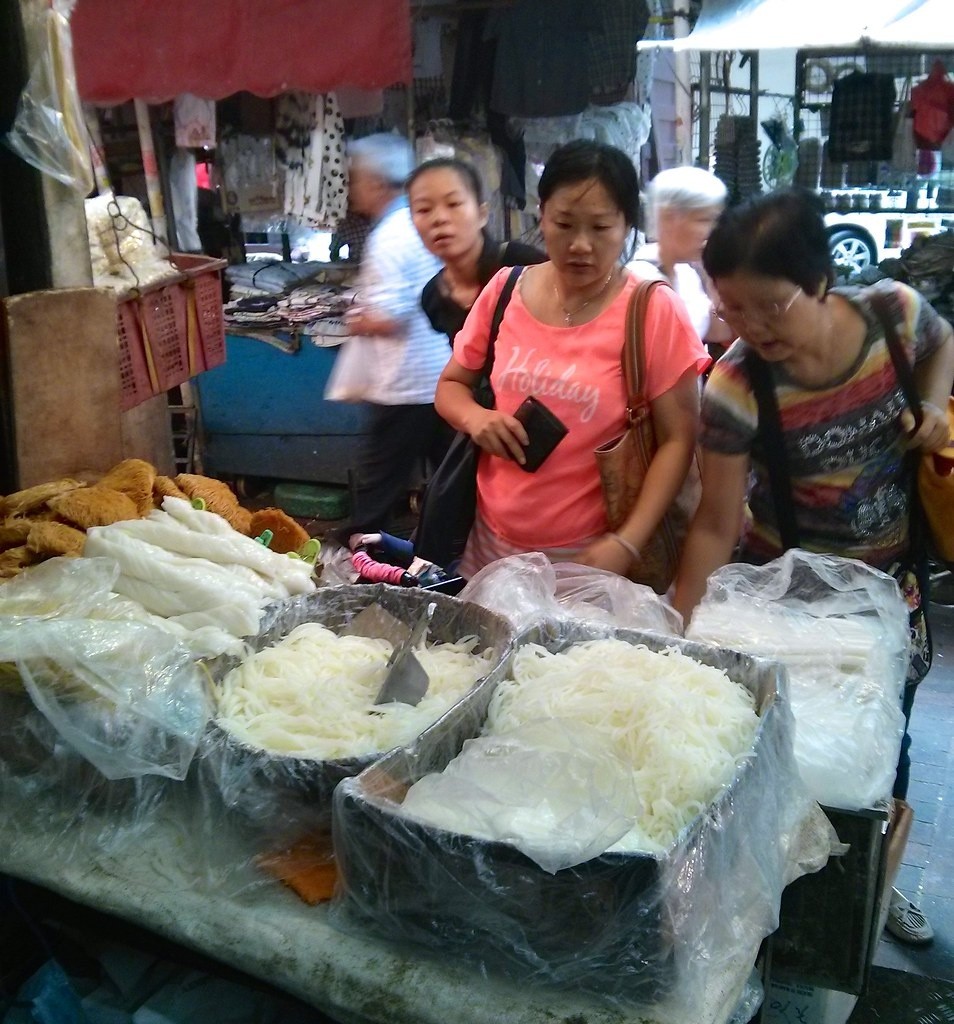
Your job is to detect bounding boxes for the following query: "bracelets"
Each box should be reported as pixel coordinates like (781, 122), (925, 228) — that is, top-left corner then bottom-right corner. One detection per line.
(605, 529), (645, 567)
(918, 396), (945, 416)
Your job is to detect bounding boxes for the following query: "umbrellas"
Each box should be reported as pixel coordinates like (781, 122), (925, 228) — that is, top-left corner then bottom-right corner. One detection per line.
(52, 1), (414, 252)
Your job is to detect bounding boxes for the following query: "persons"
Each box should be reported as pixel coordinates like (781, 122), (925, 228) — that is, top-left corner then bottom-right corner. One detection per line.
(325, 133), (954, 948)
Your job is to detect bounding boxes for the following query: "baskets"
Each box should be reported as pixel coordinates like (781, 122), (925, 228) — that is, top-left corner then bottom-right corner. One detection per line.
(118, 252), (228, 416)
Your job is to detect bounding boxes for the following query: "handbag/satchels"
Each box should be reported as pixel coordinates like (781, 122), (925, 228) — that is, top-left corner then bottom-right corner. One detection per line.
(593, 279), (704, 595)
(412, 264), (524, 574)
(918, 396), (954, 563)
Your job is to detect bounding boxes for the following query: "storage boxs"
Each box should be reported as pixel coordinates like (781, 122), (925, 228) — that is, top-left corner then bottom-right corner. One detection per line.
(108, 250), (227, 414)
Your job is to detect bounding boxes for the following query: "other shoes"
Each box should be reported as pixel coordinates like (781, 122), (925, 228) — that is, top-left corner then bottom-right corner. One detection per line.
(885, 900), (934, 942)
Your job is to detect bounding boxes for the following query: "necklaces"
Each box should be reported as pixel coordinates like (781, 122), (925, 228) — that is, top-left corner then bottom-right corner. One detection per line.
(549, 266), (609, 326)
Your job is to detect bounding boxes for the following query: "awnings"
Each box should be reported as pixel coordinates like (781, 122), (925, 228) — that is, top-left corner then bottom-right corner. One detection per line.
(639, 0), (954, 53)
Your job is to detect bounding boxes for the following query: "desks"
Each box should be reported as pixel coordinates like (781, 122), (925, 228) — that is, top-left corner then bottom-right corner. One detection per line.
(0, 689), (921, 1024)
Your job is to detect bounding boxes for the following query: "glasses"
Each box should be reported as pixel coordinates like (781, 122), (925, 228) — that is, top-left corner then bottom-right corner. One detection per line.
(713, 283), (804, 324)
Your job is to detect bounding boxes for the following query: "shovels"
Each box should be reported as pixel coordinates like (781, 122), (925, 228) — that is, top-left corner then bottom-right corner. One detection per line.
(371, 601), (439, 707)
